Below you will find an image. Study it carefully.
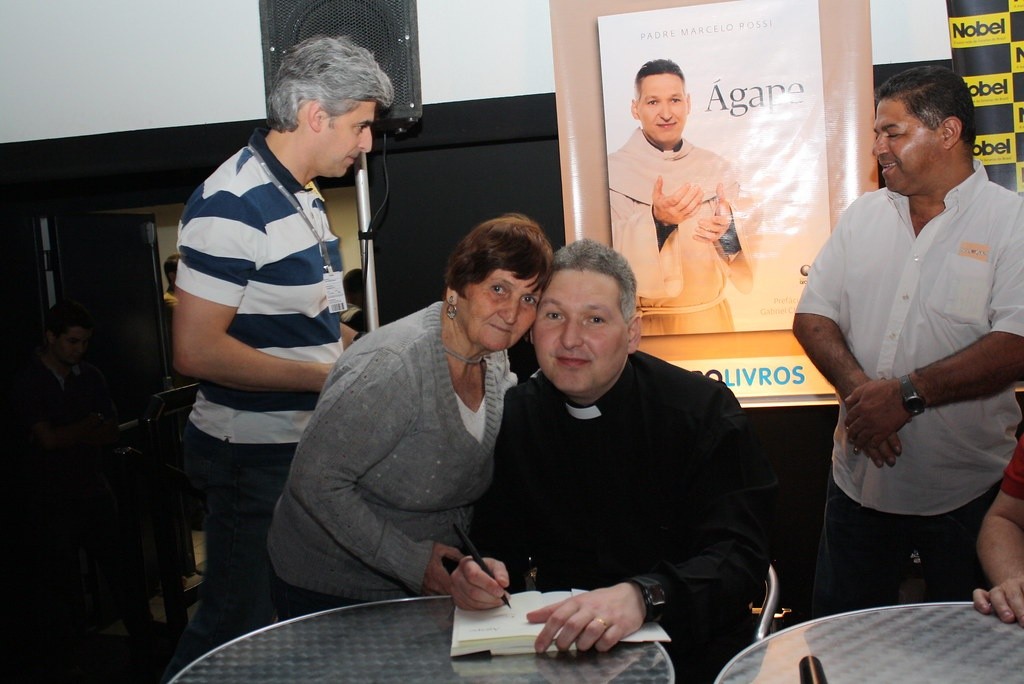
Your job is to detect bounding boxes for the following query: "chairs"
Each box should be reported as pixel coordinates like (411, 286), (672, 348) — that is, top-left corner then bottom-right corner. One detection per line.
(749, 563), (793, 643)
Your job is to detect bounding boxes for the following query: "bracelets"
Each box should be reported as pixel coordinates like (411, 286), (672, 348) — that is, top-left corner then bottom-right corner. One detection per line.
(352, 330), (368, 342)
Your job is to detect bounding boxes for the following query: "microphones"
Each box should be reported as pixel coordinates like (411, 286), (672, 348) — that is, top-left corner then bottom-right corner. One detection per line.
(798, 656), (828, 684)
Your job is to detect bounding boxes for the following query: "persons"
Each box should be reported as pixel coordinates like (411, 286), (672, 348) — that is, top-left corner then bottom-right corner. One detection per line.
(448, 236), (777, 684)
(266, 216), (554, 611)
(339, 268), (365, 331)
(608, 58), (756, 336)
(790, 67), (1024, 616)
(161, 34), (396, 684)
(973, 430), (1024, 629)
(163, 254), (180, 306)
(10, 299), (175, 684)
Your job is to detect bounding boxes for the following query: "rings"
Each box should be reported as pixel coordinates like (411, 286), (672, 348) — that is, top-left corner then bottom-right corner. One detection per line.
(593, 618), (607, 629)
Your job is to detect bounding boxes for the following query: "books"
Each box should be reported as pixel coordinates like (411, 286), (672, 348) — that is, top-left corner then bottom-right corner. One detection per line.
(450, 587), (672, 658)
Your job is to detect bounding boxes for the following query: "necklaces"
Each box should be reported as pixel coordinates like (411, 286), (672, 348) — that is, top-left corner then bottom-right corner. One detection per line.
(445, 347), (483, 364)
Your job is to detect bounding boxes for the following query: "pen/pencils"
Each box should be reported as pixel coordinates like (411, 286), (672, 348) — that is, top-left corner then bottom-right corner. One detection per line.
(452, 521), (512, 609)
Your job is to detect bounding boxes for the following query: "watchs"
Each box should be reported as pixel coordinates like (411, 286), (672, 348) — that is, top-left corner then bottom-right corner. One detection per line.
(629, 576), (666, 621)
(899, 374), (925, 415)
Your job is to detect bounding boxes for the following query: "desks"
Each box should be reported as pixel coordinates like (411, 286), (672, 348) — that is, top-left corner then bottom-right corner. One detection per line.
(711, 601), (1024, 684)
(164, 595), (676, 684)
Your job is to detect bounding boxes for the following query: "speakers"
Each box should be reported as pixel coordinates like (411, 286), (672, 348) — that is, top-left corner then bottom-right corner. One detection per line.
(257, 0), (422, 135)
